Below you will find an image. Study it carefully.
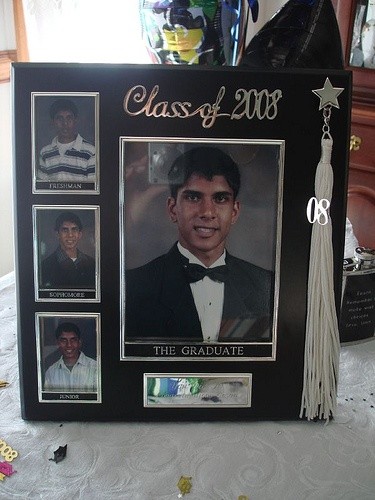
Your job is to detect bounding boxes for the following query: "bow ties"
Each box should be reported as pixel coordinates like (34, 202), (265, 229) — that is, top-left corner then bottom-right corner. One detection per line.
(181, 262), (228, 283)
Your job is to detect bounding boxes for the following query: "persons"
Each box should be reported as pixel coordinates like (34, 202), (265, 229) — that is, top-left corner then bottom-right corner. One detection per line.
(44, 322), (99, 391)
(125, 145), (272, 342)
(37, 100), (95, 182)
(40, 212), (95, 289)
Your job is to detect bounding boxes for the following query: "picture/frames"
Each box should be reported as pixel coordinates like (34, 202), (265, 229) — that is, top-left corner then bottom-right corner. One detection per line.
(11, 61), (349, 425)
